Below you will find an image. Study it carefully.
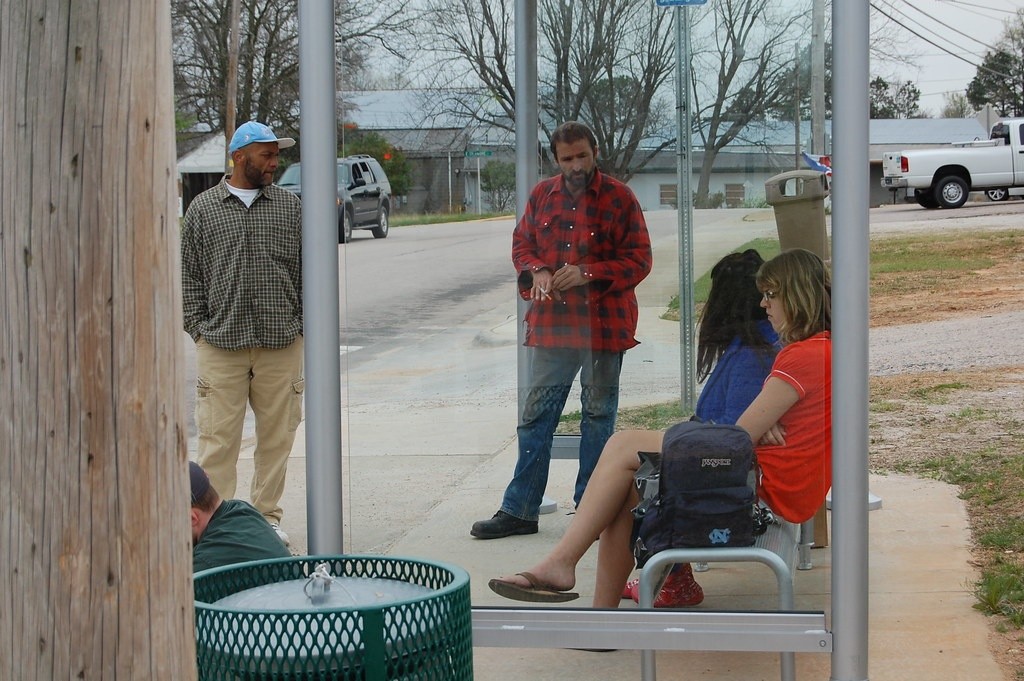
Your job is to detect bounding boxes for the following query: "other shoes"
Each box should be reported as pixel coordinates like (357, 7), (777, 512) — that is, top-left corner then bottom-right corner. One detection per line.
(631, 565), (703, 607)
(622, 577), (638, 598)
(271, 523), (289, 545)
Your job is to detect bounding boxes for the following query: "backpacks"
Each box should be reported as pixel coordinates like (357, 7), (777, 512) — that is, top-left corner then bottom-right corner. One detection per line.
(635, 415), (757, 569)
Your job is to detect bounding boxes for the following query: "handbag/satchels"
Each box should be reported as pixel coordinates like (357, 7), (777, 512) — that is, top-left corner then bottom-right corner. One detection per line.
(633, 452), (762, 500)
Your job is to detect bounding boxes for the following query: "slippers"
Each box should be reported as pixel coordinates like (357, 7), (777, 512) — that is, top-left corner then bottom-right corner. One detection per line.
(489, 573), (579, 603)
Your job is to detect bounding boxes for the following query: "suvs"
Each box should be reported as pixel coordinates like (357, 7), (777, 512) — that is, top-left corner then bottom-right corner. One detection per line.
(274, 153), (393, 243)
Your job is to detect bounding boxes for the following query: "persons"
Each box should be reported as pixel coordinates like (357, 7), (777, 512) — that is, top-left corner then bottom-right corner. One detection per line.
(189, 458), (304, 617)
(470, 122), (653, 543)
(181, 121), (301, 546)
(623, 249), (788, 607)
(487, 247), (832, 653)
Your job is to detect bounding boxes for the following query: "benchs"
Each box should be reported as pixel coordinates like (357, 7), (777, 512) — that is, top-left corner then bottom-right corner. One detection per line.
(639, 513), (814, 681)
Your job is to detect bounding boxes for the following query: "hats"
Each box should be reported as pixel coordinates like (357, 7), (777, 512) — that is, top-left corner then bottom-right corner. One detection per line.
(189, 460), (209, 503)
(228, 121), (296, 156)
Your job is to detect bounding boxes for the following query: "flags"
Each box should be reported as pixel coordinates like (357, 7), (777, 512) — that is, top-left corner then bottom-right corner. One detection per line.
(802, 152), (832, 175)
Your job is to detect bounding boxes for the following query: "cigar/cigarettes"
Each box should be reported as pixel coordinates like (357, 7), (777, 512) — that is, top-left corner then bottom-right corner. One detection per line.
(536, 284), (552, 302)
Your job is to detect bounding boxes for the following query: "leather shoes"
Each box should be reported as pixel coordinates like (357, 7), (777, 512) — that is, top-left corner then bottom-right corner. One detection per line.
(470, 510), (535, 538)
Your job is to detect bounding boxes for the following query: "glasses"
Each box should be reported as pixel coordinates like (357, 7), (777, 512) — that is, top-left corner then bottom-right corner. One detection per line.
(763, 291), (780, 301)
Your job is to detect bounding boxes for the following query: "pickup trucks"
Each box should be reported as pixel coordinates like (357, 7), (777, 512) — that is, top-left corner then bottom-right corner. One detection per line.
(880, 116), (1024, 209)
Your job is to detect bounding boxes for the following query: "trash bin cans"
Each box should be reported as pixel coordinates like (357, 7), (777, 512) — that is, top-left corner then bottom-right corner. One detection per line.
(192, 553), (474, 681)
(764, 170), (830, 262)
